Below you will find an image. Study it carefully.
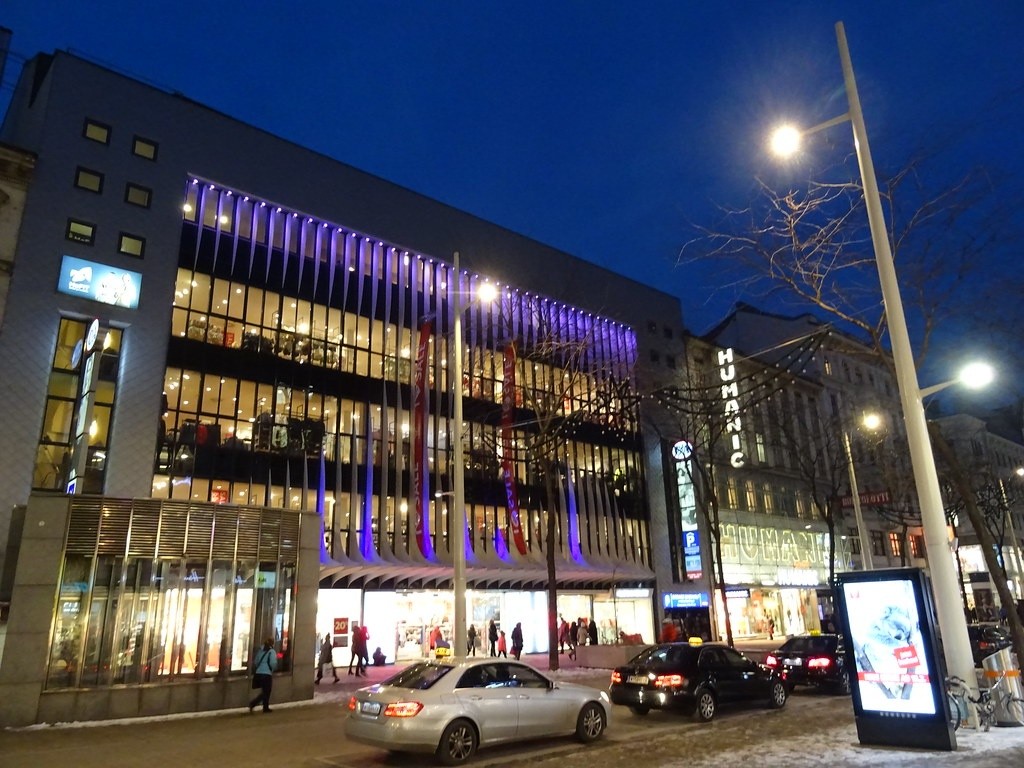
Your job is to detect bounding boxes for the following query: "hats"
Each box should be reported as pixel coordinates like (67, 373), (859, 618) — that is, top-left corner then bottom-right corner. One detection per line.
(581, 622), (586, 626)
(589, 616), (594, 621)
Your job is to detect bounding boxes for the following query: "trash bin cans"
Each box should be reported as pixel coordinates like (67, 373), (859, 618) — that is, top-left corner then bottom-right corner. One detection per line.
(980, 646), (1024, 727)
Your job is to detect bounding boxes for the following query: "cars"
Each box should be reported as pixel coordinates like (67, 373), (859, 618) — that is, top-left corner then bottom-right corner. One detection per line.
(343, 657), (611, 766)
(766, 635), (851, 696)
(608, 642), (789, 722)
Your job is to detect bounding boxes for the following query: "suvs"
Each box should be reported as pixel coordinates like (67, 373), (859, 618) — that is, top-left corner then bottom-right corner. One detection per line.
(966, 625), (1011, 668)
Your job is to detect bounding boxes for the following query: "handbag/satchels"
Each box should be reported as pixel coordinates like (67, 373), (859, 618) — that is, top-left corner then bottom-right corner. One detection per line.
(251, 671), (260, 689)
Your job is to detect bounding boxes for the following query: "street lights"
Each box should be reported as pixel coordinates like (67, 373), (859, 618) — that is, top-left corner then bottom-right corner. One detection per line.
(838, 414), (881, 570)
(452, 248), (498, 661)
(761, 18), (988, 734)
(997, 467), (1024, 599)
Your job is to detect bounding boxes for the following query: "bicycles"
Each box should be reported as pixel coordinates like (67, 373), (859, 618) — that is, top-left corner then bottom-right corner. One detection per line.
(944, 671), (1024, 731)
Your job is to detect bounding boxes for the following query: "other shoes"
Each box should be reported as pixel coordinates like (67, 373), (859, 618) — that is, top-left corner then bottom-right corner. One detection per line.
(315, 681), (320, 685)
(334, 677), (339, 682)
(263, 707), (272, 712)
(560, 648), (564, 654)
(248, 704), (252, 712)
(568, 654), (572, 660)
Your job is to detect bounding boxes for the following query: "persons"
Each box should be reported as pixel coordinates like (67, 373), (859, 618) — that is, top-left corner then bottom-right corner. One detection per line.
(557, 616), (599, 661)
(427, 621), (524, 660)
(766, 614), (777, 640)
(247, 638), (277, 713)
(347, 624), (369, 678)
(373, 646), (385, 663)
(316, 633), (339, 686)
(677, 469), (685, 502)
